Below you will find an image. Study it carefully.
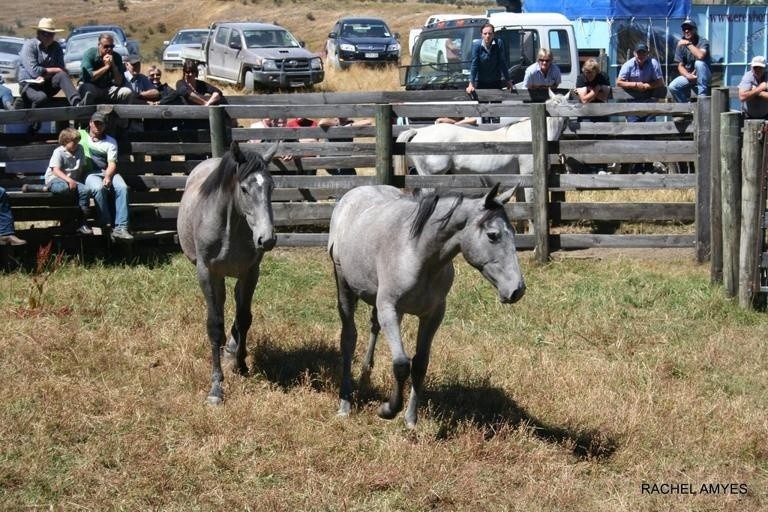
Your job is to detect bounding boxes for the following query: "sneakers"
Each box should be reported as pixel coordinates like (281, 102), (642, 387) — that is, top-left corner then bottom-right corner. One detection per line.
(79, 92), (94, 106)
(110, 232), (116, 243)
(13, 98), (24, 110)
(22, 184), (28, 193)
(673, 117), (685, 122)
(112, 228), (134, 240)
(75, 224), (93, 234)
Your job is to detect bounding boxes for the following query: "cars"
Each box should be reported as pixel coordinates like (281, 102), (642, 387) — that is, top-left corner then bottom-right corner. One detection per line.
(325, 16), (401, 71)
(64, 32), (129, 75)
(0, 36), (22, 82)
(57, 39), (66, 60)
(162, 28), (214, 71)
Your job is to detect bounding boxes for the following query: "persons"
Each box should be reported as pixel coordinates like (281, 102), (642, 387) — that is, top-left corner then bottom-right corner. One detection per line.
(314, 116), (374, 175)
(284, 117), (322, 175)
(737, 55), (768, 118)
(0, 13), (224, 248)
(248, 118), (275, 145)
(573, 58), (611, 140)
(465, 24), (516, 123)
(522, 47), (561, 91)
(614, 43), (665, 122)
(666, 18), (713, 102)
(258, 115), (299, 161)
(445, 38), (462, 72)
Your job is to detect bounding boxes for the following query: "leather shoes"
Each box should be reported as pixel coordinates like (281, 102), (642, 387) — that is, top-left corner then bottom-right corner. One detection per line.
(0, 235), (27, 245)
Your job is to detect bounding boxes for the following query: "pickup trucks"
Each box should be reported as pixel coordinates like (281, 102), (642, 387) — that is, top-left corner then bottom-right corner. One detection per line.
(66, 24), (142, 73)
(182, 21), (325, 92)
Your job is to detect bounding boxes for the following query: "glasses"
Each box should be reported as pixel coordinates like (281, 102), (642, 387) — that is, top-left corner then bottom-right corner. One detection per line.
(149, 74), (160, 77)
(682, 27), (693, 32)
(185, 71), (195, 74)
(539, 60), (549, 63)
(41, 33), (54, 37)
(101, 43), (115, 49)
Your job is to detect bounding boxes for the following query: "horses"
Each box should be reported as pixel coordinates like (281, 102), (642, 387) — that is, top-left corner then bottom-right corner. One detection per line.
(325, 180), (528, 428)
(394, 84), (584, 239)
(175, 135), (282, 406)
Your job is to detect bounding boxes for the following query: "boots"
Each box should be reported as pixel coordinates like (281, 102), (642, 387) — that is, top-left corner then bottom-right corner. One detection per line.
(116, 125), (130, 154)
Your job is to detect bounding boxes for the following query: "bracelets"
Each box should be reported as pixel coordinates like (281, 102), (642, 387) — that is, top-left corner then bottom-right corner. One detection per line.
(685, 41), (693, 48)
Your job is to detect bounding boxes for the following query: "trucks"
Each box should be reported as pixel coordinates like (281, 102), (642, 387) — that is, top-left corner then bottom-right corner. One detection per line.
(402, 2), (718, 91)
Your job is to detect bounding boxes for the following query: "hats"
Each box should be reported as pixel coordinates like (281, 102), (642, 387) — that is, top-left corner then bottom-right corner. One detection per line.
(126, 55), (140, 64)
(91, 112), (106, 122)
(751, 56), (767, 68)
(31, 18), (65, 33)
(681, 20), (696, 27)
(635, 44), (648, 53)
